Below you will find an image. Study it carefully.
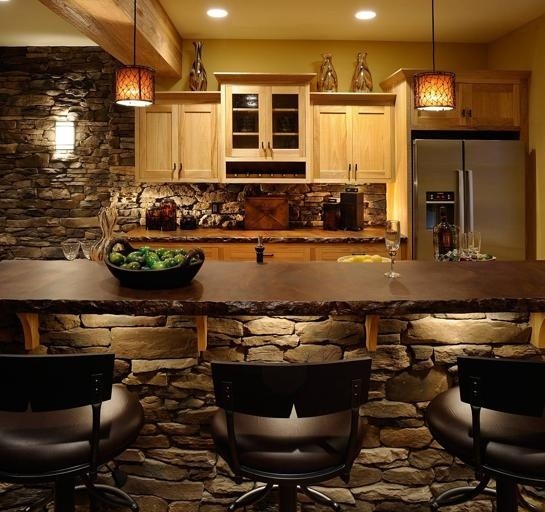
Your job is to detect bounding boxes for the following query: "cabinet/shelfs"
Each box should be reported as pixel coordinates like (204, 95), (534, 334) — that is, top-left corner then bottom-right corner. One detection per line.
(307, 90), (398, 185)
(221, 238), (314, 266)
(380, 66), (534, 140)
(211, 71), (319, 186)
(311, 239), (408, 265)
(134, 87), (221, 185)
(118, 238), (224, 264)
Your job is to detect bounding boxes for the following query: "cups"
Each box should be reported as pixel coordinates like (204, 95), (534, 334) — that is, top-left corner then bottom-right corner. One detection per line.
(60, 242), (79, 261)
(80, 240), (97, 259)
(459, 232), (481, 261)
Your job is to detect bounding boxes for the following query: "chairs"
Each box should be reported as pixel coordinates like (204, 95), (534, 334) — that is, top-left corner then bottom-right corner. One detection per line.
(203, 354), (376, 511)
(0, 346), (151, 511)
(414, 352), (545, 512)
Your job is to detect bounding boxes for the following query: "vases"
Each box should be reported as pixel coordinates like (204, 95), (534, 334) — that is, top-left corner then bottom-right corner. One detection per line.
(351, 50), (374, 94)
(187, 37), (209, 93)
(316, 51), (340, 92)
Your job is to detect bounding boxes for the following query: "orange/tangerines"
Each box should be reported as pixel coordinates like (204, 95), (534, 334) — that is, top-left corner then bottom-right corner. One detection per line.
(109, 246), (197, 270)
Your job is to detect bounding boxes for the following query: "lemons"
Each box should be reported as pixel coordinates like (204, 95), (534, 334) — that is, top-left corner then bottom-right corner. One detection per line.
(342, 254), (389, 264)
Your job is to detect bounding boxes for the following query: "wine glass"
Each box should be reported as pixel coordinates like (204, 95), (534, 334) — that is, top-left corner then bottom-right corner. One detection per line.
(384, 219), (401, 279)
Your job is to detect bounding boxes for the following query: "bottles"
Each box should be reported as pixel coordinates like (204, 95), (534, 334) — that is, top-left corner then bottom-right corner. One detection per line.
(255, 235), (264, 264)
(433, 207), (456, 260)
(145, 198), (196, 231)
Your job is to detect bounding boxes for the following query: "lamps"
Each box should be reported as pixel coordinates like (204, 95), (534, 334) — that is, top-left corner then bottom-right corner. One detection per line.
(111, 0), (158, 108)
(411, 0), (457, 114)
(48, 109), (84, 160)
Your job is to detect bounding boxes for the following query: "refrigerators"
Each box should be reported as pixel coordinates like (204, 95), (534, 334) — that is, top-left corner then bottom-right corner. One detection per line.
(412, 138), (526, 260)
